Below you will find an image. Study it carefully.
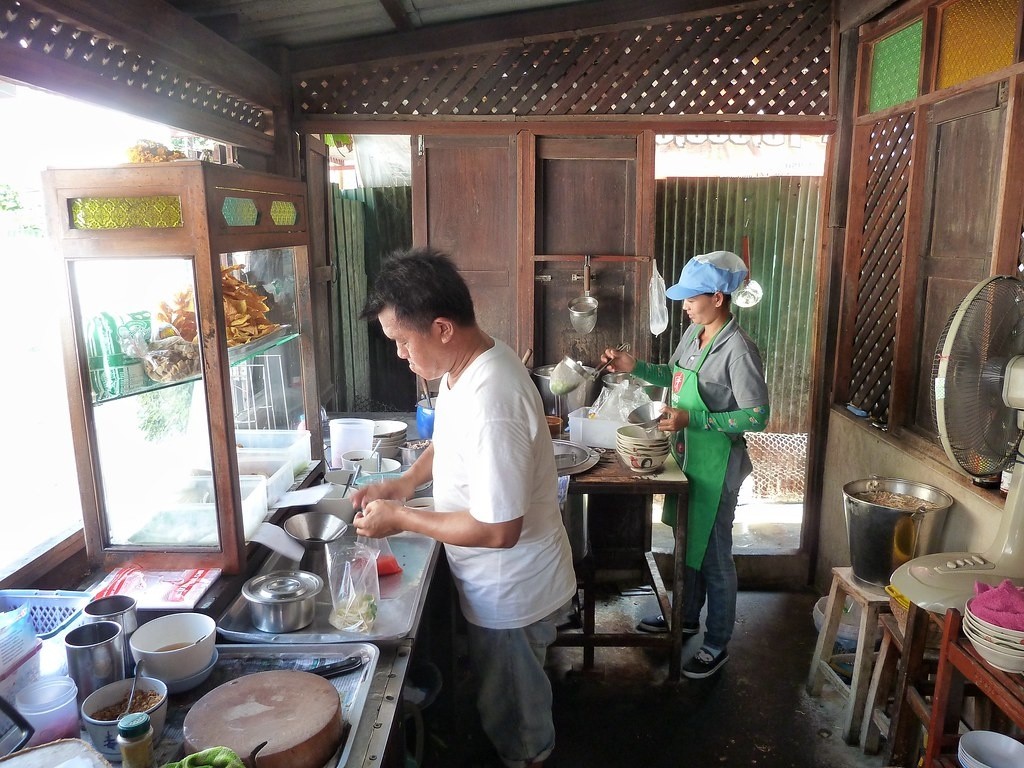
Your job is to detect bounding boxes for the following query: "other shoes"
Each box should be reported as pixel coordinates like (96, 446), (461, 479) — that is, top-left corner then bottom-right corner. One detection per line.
(639, 614), (700, 634)
(682, 647), (729, 679)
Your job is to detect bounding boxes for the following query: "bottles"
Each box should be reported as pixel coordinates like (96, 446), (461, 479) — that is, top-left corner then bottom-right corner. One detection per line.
(116, 712), (158, 768)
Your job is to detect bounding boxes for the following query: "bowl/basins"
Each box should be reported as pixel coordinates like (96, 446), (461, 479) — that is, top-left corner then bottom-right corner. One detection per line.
(129, 613), (216, 679)
(404, 496), (434, 511)
(616, 426), (672, 472)
(341, 449), (378, 469)
(372, 419), (408, 459)
(962, 596), (1024, 674)
(241, 570), (323, 632)
(352, 458), (401, 474)
(81, 676), (168, 761)
(16, 676), (78, 731)
(416, 397), (437, 439)
(133, 648), (218, 692)
(310, 470), (402, 524)
(626, 400), (668, 429)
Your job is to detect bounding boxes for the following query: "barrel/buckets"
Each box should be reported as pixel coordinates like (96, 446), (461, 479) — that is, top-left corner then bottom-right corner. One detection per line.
(534, 365), (600, 423)
(813, 595), (883, 679)
(841, 475), (954, 597)
(602, 373), (669, 405)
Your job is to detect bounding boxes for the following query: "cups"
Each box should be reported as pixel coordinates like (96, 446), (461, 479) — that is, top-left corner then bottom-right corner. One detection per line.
(82, 595), (138, 673)
(329, 417), (375, 470)
(64, 620), (125, 705)
(399, 439), (430, 464)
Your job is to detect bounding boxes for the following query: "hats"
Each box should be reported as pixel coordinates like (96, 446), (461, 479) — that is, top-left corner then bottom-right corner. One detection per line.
(665, 250), (749, 300)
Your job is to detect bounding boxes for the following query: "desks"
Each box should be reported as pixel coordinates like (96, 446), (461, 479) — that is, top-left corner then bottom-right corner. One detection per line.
(556, 432), (690, 682)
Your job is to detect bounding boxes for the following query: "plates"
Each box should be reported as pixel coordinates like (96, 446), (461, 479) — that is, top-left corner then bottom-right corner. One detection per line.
(957, 730), (1024, 768)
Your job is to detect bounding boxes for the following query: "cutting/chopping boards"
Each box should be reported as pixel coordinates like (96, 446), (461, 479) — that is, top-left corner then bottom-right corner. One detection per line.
(182, 671), (342, 768)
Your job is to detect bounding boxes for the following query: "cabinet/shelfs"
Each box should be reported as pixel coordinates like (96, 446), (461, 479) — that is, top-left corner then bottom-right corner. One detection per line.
(38, 159), (326, 577)
(883, 600), (1024, 768)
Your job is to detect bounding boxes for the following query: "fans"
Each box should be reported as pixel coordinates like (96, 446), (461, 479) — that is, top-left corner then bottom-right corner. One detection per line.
(890, 274), (1024, 616)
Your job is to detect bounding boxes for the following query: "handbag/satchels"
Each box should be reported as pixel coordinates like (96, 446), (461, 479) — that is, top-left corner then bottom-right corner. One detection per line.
(649, 259), (669, 337)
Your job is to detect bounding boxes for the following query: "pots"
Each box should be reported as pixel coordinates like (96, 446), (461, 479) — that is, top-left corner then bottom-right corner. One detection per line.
(552, 438), (606, 476)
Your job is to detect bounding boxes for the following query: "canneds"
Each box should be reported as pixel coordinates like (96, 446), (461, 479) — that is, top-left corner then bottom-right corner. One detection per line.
(116, 712), (159, 768)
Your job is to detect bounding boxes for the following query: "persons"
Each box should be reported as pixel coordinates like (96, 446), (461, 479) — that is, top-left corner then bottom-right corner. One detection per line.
(601, 251), (770, 679)
(349, 249), (577, 768)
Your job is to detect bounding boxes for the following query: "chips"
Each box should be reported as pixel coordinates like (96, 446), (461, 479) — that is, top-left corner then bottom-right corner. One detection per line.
(156, 266), (278, 347)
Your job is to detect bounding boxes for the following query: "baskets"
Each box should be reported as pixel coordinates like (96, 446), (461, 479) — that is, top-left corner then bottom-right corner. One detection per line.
(0, 638), (43, 736)
(0, 589), (92, 675)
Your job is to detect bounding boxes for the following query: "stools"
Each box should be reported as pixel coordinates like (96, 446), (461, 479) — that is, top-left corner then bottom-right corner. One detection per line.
(807, 566), (939, 755)
